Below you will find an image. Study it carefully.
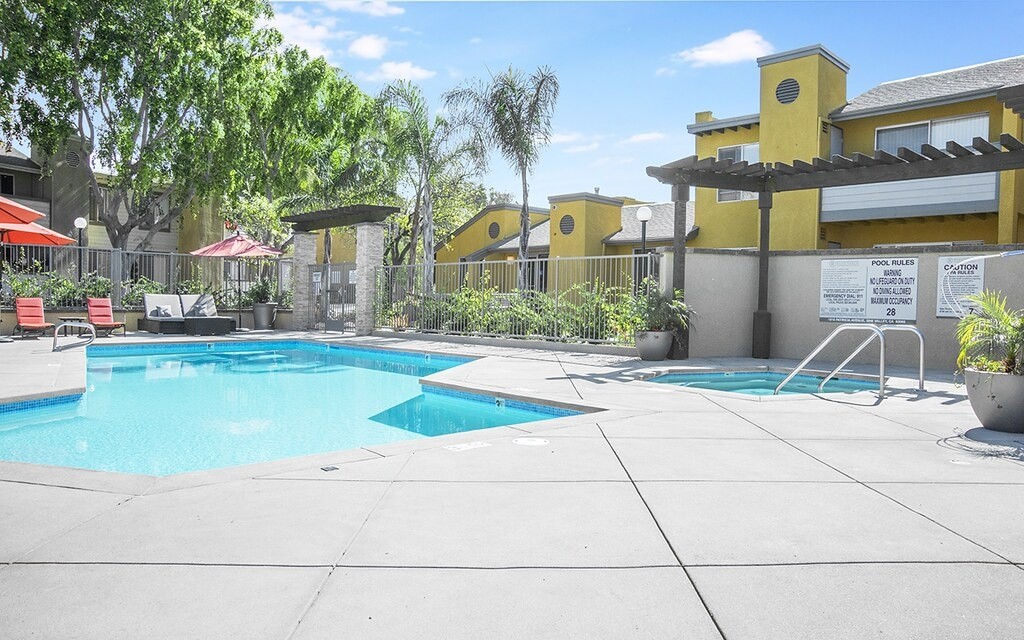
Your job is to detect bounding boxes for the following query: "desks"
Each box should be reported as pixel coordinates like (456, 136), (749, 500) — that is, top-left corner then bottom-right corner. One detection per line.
(58, 316), (87, 337)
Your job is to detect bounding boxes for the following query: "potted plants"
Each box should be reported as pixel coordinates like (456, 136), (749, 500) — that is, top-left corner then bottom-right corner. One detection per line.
(247, 279), (278, 330)
(627, 285), (704, 360)
(951, 288), (1024, 434)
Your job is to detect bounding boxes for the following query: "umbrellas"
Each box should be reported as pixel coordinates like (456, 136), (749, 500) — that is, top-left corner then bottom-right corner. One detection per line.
(0, 222), (78, 305)
(0, 196), (46, 224)
(190, 231), (288, 328)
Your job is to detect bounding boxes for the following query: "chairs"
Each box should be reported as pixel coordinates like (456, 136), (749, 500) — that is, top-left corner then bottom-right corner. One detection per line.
(84, 298), (127, 338)
(137, 294), (236, 335)
(12, 297), (56, 340)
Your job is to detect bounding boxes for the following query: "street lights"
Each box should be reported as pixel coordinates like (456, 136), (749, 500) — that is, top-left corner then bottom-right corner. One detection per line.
(73, 217), (88, 282)
(637, 207), (652, 253)
